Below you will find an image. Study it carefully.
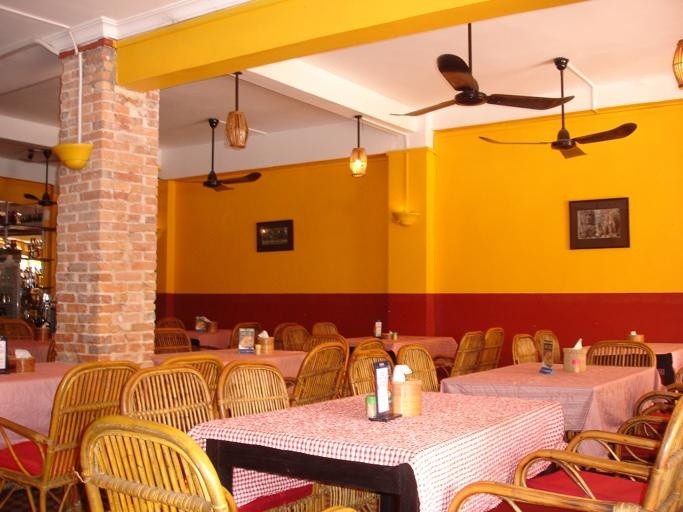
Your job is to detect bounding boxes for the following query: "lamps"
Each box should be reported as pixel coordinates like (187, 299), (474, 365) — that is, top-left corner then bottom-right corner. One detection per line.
(51, 142), (93, 169)
(349, 114), (367, 177)
(392, 210), (421, 226)
(672, 39), (683, 87)
(225, 72), (249, 148)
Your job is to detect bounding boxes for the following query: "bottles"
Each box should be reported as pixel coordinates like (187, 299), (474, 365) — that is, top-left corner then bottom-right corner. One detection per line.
(255, 344), (260, 354)
(0, 324), (7, 374)
(366, 395), (376, 418)
(383, 330), (398, 339)
(571, 359), (579, 373)
(0, 208), (56, 327)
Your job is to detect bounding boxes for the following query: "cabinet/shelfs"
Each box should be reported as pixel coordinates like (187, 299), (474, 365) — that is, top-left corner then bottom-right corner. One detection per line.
(0, 200), (58, 311)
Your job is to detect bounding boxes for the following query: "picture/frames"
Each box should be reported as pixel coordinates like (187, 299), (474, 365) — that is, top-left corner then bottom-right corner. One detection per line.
(256, 219), (294, 252)
(569, 197), (630, 249)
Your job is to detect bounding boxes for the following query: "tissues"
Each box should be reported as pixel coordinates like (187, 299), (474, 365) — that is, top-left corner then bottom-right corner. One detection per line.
(200, 316), (217, 334)
(391, 365), (422, 415)
(257, 330), (275, 352)
(563, 338), (591, 371)
(8, 348), (35, 372)
(625, 331), (644, 343)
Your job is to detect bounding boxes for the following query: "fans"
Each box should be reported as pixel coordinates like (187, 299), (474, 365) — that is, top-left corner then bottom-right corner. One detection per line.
(24, 147), (59, 206)
(479, 57), (637, 159)
(155, 118), (262, 191)
(390, 23), (575, 117)
